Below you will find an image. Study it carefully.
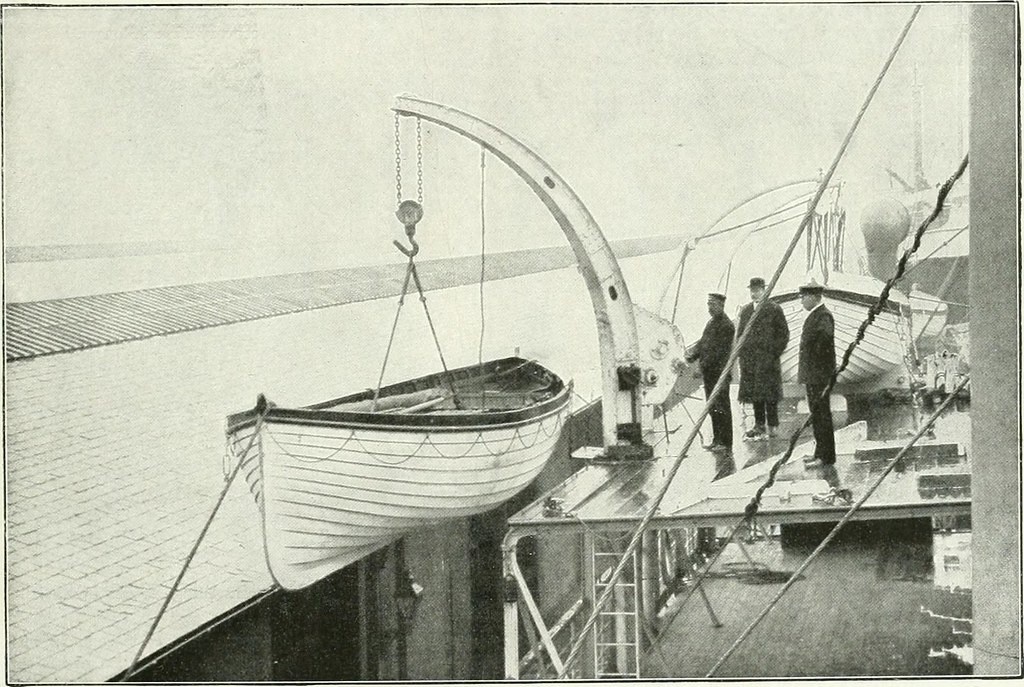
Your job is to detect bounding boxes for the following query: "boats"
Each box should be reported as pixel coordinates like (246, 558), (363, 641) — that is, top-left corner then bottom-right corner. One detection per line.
(903, 290), (948, 342)
(222, 348), (574, 587)
(760, 267), (911, 391)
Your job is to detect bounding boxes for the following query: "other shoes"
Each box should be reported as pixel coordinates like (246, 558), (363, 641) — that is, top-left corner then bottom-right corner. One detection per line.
(746, 425), (766, 437)
(702, 443), (733, 451)
(802, 455), (835, 468)
(768, 427), (777, 437)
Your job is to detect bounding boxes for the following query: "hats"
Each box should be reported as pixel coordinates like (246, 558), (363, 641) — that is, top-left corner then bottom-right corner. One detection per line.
(707, 293), (726, 301)
(794, 286), (823, 295)
(747, 278), (768, 288)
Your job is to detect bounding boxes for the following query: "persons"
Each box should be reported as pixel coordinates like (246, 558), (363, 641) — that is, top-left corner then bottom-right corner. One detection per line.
(797, 283), (840, 469)
(684, 292), (737, 453)
(734, 277), (791, 442)
